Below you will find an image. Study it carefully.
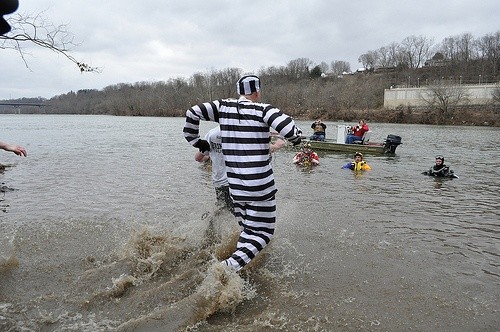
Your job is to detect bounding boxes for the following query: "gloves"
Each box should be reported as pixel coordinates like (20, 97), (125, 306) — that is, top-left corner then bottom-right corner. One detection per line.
(194, 139), (211, 153)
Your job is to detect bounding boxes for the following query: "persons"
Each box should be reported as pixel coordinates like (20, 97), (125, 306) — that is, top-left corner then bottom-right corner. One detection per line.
(182, 74), (303, 273)
(292, 141), (320, 166)
(341, 152), (371, 170)
(421, 155), (459, 178)
(347, 120), (369, 144)
(309, 120), (327, 141)
(195, 123), (285, 213)
(0, 140), (27, 157)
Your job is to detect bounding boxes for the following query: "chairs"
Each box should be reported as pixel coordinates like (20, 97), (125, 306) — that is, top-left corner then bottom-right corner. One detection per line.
(352, 131), (372, 145)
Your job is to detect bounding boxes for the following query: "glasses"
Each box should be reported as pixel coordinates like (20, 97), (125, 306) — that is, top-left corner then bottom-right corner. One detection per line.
(435, 156), (444, 159)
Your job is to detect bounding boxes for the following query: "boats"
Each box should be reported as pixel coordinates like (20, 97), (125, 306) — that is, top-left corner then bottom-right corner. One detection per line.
(269, 123), (403, 158)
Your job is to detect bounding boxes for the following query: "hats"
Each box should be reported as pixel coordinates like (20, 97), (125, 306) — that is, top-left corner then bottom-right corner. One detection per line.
(236, 75), (260, 94)
(354, 152), (363, 160)
